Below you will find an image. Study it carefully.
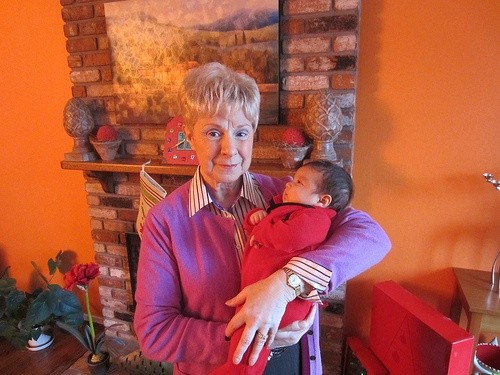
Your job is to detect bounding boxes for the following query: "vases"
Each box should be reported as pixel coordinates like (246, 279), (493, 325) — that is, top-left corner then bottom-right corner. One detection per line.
(273, 143), (312, 170)
(90, 137), (122, 163)
(86, 350), (111, 375)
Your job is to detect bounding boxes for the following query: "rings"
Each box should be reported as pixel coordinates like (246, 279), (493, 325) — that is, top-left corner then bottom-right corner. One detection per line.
(257, 331), (268, 340)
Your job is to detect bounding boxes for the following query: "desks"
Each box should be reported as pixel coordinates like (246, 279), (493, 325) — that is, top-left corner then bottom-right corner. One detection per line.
(450, 266), (500, 375)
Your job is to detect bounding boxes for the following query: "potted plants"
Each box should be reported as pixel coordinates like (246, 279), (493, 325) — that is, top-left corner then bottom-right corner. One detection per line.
(0, 259), (85, 351)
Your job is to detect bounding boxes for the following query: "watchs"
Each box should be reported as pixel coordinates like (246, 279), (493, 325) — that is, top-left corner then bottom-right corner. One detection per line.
(282, 266), (301, 296)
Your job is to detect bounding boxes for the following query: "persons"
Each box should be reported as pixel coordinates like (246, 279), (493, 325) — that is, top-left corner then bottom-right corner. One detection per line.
(236, 160), (354, 333)
(132, 64), (392, 375)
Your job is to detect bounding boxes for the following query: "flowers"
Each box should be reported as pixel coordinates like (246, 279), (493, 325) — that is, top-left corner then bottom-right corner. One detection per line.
(55, 261), (126, 363)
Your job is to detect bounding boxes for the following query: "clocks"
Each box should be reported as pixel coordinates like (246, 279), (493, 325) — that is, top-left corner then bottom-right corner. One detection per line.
(163, 115), (201, 165)
